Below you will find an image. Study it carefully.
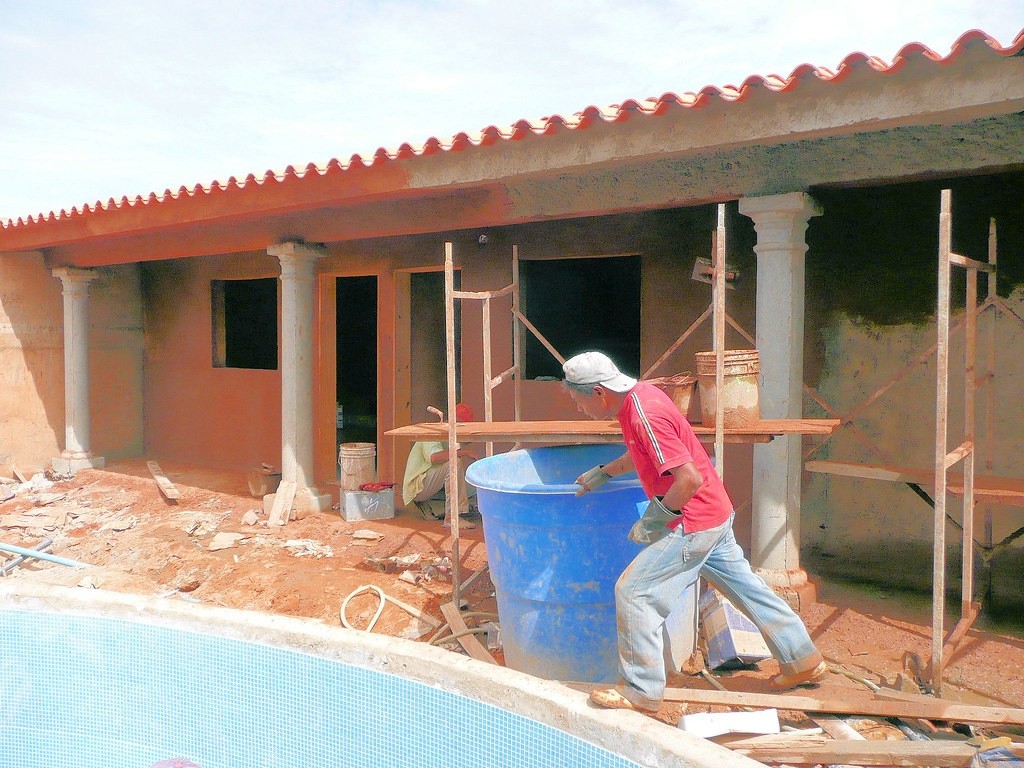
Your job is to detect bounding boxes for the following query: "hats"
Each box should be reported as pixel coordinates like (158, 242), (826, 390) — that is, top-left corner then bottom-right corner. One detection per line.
(456, 403), (473, 421)
(563, 352), (637, 393)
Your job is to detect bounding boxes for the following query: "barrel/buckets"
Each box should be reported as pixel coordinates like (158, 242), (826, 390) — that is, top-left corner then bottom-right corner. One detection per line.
(644, 370), (698, 423)
(694, 349), (761, 429)
(338, 443), (377, 491)
(463, 441), (715, 685)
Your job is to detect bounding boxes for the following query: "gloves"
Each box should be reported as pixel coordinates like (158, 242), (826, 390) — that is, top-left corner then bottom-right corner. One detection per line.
(573, 464), (613, 497)
(628, 495), (683, 544)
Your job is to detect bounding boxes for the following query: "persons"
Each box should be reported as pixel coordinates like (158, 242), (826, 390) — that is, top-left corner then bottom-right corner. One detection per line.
(402, 404), (481, 529)
(563, 351), (828, 709)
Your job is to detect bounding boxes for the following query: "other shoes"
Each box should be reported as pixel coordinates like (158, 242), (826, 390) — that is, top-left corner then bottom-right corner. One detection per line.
(768, 661), (830, 690)
(590, 688), (658, 717)
(414, 500), (438, 520)
(444, 517), (476, 529)
